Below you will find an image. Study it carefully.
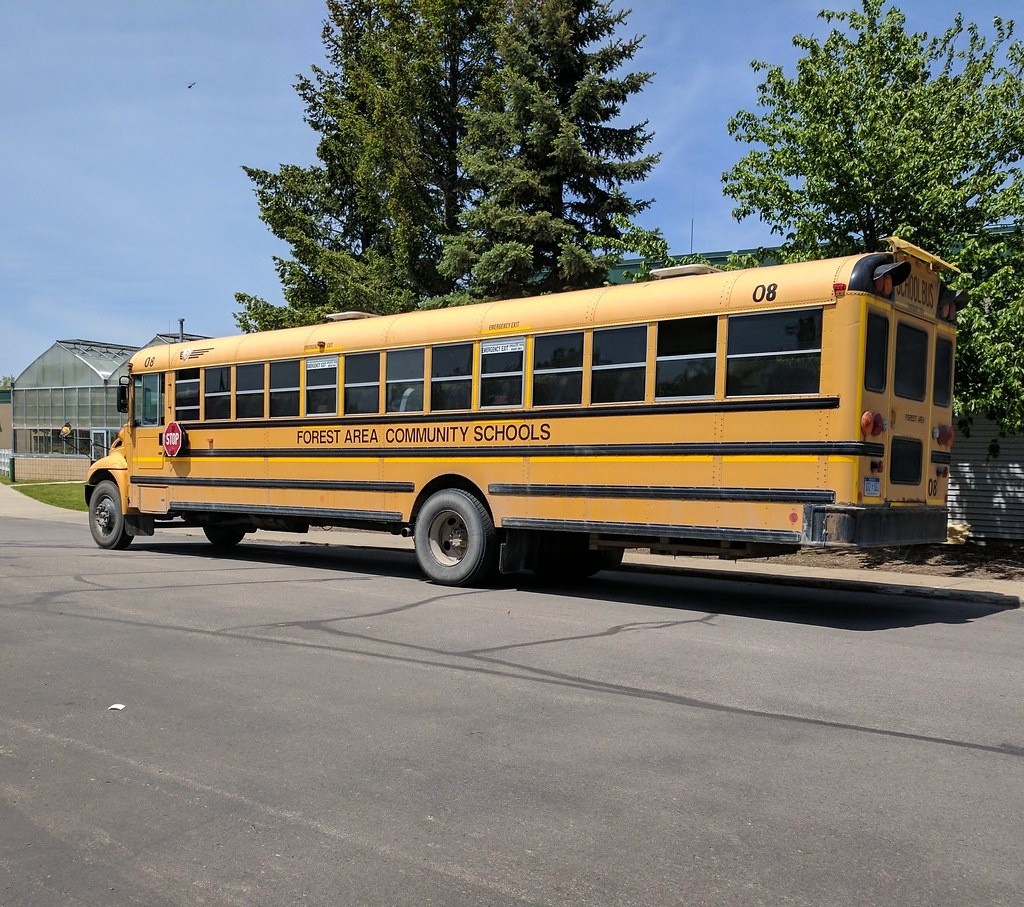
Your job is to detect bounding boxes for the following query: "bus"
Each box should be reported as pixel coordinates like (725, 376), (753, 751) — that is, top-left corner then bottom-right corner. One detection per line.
(59, 227), (971, 587)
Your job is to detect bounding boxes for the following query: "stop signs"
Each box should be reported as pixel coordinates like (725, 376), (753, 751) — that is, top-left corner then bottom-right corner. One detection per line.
(164, 420), (182, 457)
